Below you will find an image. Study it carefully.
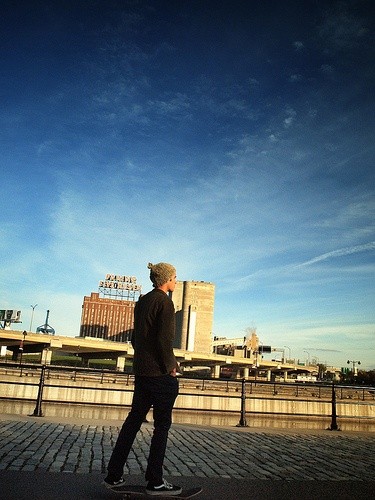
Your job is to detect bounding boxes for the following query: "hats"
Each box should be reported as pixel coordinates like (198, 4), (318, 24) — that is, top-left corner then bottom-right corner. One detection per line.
(148, 262), (176, 288)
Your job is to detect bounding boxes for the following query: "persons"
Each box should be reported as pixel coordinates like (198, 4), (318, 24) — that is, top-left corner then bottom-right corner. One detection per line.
(102, 262), (183, 496)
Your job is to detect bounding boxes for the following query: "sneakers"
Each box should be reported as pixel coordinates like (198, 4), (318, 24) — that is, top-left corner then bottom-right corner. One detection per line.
(105, 477), (125, 487)
(146, 478), (183, 496)
